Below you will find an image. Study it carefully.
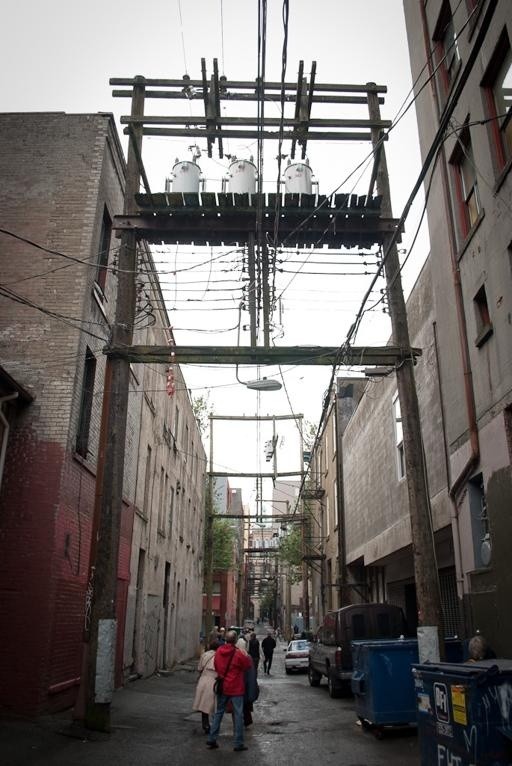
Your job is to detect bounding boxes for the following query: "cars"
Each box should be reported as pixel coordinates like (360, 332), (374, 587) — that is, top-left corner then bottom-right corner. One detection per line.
(282, 640), (310, 674)
(244, 618), (255, 631)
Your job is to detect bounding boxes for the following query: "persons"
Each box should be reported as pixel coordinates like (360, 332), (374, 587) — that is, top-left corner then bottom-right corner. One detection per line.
(463, 636), (496, 665)
(209, 626), (276, 676)
(231, 638), (258, 728)
(192, 640), (224, 733)
(206, 631), (253, 752)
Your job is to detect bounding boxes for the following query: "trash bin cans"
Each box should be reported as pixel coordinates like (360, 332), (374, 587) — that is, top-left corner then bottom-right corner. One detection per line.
(348, 634), (512, 766)
(228, 626), (243, 636)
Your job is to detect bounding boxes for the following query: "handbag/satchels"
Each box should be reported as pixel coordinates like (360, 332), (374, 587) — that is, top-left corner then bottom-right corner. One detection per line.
(213, 649), (235, 695)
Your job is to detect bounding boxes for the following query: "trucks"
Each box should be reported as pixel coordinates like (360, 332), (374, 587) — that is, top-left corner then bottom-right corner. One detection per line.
(308, 603), (412, 697)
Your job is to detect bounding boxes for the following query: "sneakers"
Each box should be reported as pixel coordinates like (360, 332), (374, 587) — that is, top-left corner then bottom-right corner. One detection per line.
(233, 744), (248, 752)
(205, 740), (219, 750)
